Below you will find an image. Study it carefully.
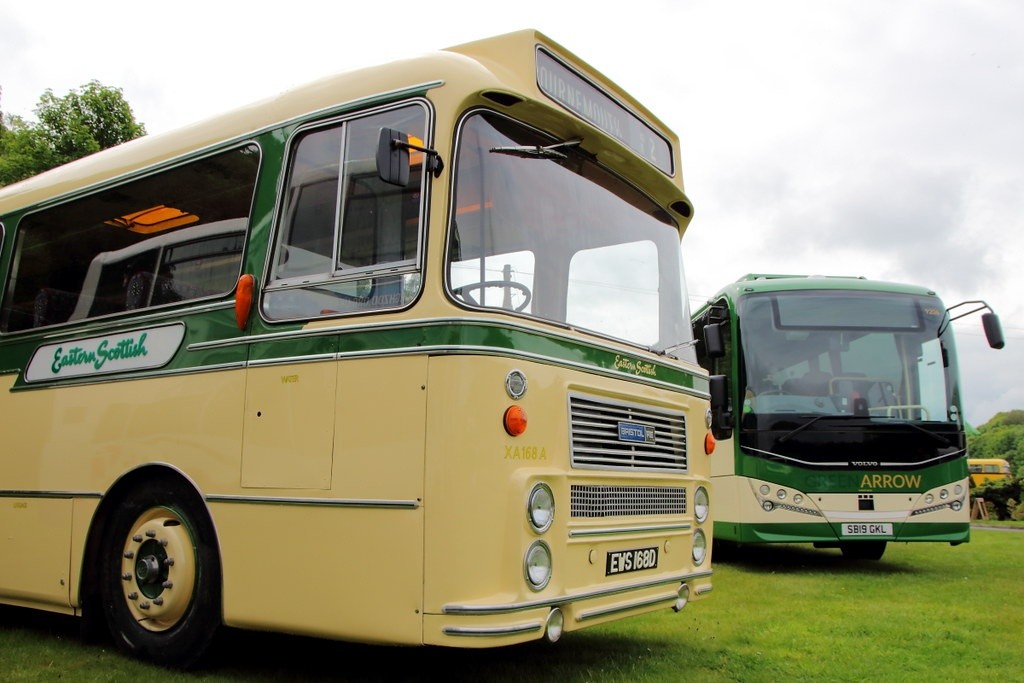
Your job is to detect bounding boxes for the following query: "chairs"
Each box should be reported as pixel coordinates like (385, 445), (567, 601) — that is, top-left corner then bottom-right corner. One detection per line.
(783, 371), (903, 419)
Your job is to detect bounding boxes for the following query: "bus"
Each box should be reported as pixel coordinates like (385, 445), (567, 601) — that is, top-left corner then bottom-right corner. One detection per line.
(967, 458), (1012, 488)
(0, 27), (734, 668)
(686, 271), (1006, 565)
